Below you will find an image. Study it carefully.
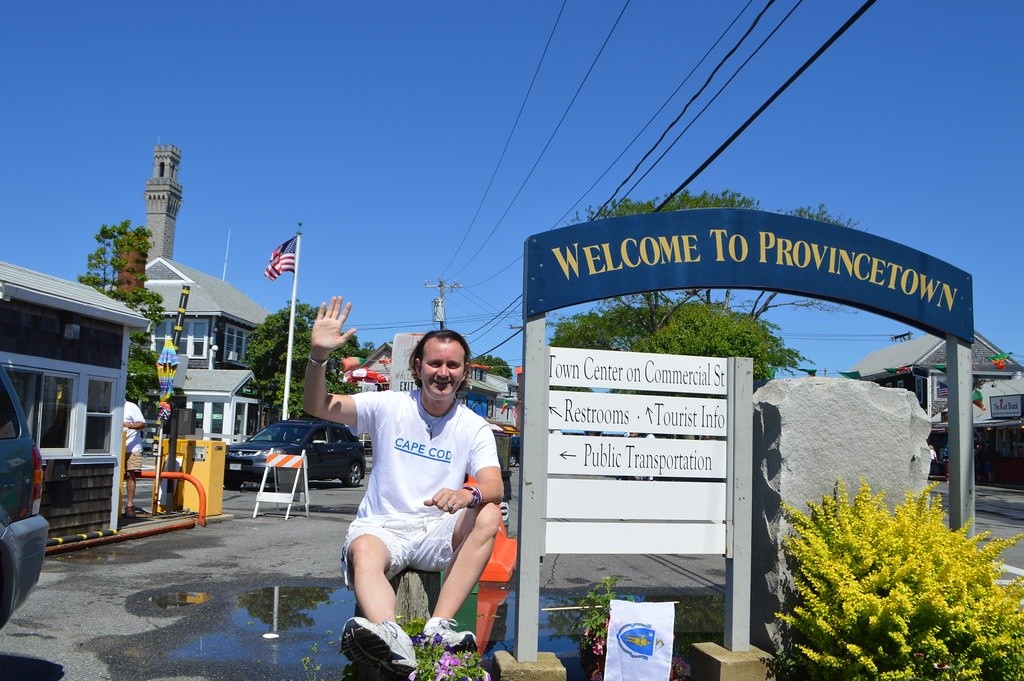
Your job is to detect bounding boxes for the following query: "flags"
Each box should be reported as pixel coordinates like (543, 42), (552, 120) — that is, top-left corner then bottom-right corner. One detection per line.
(264, 234), (298, 281)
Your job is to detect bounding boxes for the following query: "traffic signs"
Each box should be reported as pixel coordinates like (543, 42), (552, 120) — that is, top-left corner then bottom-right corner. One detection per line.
(548, 434), (727, 480)
(549, 391), (728, 438)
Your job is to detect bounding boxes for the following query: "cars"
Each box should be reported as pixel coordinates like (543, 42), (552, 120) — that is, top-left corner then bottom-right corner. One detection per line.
(0, 363), (50, 634)
(509, 436), (520, 466)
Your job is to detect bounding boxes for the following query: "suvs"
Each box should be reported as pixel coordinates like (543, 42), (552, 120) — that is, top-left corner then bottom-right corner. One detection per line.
(223, 418), (367, 490)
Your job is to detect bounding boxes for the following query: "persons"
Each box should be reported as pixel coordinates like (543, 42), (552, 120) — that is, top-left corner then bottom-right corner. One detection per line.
(122, 399), (147, 518)
(302, 294), (505, 676)
(929, 443), (939, 474)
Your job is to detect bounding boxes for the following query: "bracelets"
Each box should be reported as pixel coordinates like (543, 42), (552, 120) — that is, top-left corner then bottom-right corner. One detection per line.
(462, 485), (483, 509)
(309, 354), (331, 368)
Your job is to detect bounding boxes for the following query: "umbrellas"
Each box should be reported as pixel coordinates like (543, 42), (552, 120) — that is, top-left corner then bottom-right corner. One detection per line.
(346, 367), (387, 447)
(151, 338), (180, 517)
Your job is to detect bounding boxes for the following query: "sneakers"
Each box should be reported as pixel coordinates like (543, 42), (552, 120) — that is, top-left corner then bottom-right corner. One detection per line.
(125, 505), (136, 518)
(421, 617), (479, 655)
(342, 614), (418, 677)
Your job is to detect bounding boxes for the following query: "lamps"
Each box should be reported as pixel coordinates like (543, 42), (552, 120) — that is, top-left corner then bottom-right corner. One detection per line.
(211, 344), (219, 352)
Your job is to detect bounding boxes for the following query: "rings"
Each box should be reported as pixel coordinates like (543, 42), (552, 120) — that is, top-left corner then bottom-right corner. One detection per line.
(446, 502), (453, 511)
